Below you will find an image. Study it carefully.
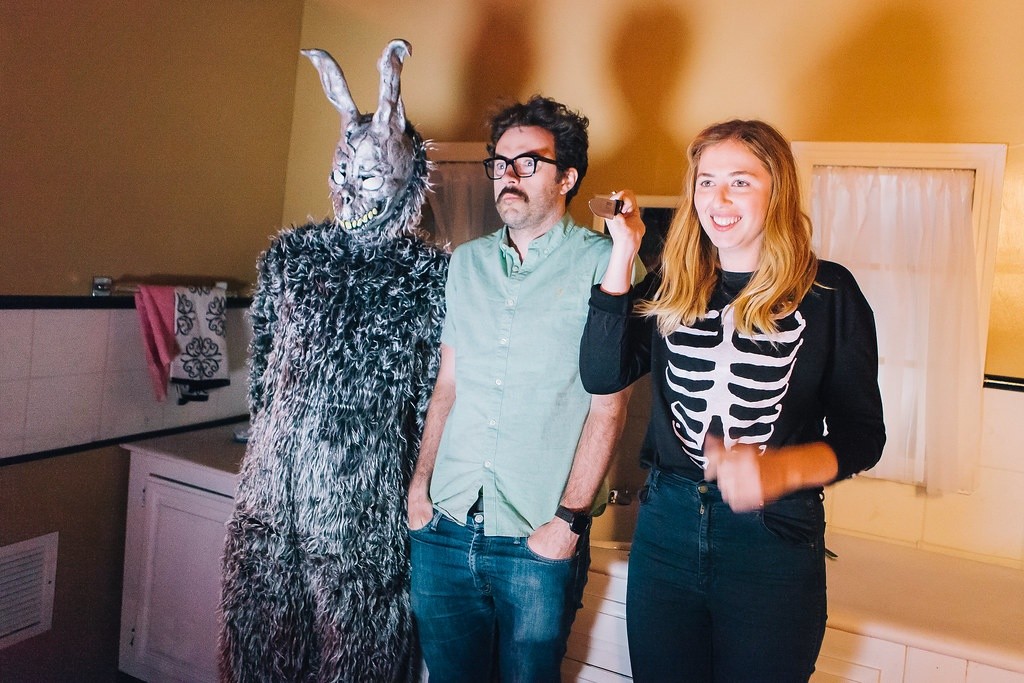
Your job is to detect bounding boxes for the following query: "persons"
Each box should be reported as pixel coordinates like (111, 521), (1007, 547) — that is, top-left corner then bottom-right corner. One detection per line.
(406, 93), (648, 683)
(579, 120), (887, 683)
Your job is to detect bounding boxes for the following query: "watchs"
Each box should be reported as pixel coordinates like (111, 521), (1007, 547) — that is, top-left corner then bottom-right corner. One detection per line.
(554, 505), (593, 536)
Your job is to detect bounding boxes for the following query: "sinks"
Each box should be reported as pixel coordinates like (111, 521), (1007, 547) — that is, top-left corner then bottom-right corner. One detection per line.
(588, 505), (638, 543)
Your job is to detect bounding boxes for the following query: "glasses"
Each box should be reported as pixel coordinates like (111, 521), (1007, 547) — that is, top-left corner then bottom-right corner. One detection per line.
(482, 154), (562, 180)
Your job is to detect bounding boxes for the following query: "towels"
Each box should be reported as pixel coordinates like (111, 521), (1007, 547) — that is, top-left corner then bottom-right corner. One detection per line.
(134, 281), (231, 406)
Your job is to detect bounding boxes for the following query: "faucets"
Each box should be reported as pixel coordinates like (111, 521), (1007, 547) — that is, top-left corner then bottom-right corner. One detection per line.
(609, 487), (635, 506)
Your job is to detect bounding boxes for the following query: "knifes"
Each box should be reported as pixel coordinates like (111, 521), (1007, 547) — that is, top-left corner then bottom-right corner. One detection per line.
(588, 198), (624, 220)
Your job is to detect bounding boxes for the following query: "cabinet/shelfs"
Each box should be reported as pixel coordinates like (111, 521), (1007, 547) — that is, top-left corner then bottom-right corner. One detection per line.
(118, 456), (1024, 683)
